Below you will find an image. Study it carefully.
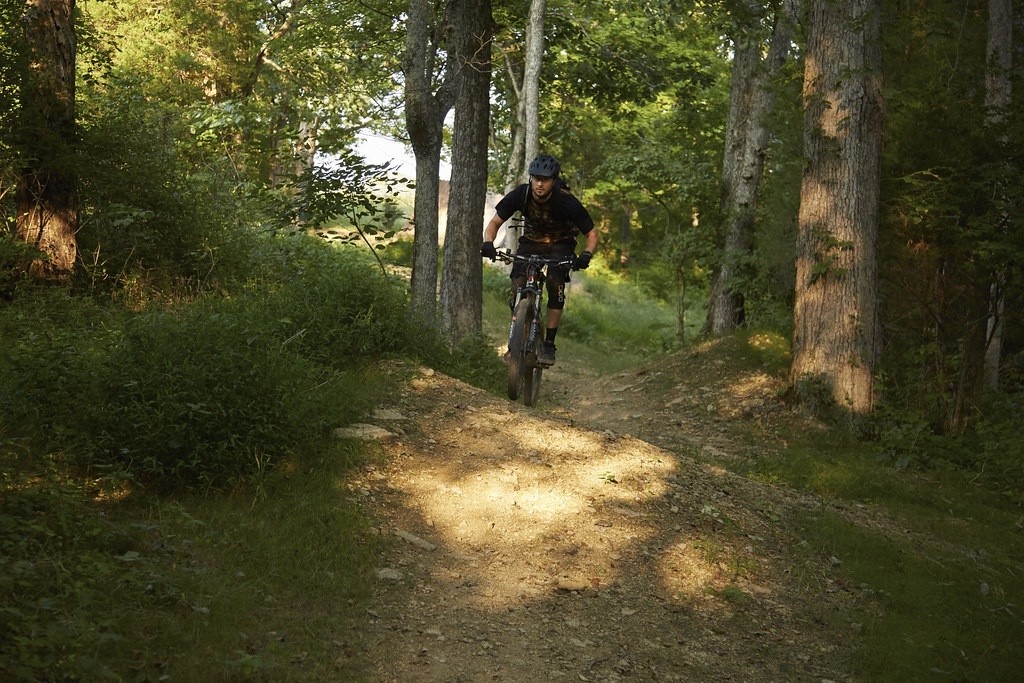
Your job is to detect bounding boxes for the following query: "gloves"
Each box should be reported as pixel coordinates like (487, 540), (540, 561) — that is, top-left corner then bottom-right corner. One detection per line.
(572, 251), (592, 271)
(481, 242), (497, 262)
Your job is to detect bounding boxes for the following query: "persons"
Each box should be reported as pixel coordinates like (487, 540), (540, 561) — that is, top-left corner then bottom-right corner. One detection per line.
(482, 155), (599, 365)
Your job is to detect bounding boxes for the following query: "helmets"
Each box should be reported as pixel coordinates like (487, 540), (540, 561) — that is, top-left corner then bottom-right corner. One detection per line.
(528, 154), (560, 176)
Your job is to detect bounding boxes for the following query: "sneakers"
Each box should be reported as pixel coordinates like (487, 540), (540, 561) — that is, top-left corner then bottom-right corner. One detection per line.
(539, 339), (557, 365)
